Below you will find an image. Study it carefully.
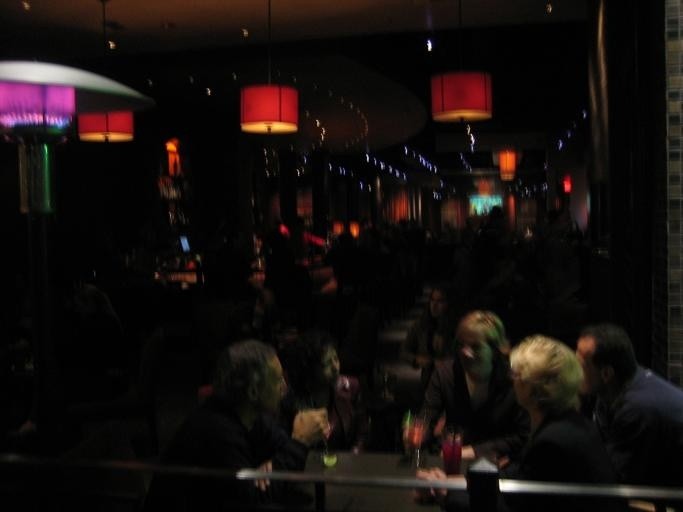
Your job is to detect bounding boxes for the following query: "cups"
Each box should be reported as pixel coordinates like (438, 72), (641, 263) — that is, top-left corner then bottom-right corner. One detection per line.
(403, 447), (427, 474)
(440, 426), (464, 475)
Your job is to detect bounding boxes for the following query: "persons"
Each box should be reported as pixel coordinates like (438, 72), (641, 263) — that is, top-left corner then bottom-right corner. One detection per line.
(0, 206), (682, 512)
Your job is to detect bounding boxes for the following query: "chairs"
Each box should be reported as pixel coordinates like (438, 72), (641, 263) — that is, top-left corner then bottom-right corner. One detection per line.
(61, 327), (170, 457)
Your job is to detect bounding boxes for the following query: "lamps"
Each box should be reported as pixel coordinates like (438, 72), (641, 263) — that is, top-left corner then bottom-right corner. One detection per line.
(75, 1), (137, 142)
(236, 0), (302, 135)
(431, 0), (496, 124)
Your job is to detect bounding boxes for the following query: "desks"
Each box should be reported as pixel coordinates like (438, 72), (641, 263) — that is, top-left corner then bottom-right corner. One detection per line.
(255, 447), (495, 512)
